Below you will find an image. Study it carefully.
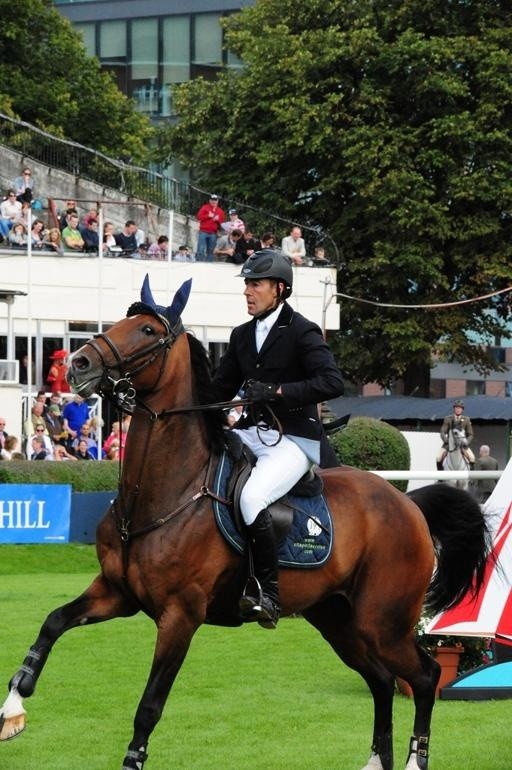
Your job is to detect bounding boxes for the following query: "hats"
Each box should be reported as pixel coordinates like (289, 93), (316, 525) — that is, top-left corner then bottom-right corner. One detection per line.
(210, 194), (217, 200)
(48, 350), (67, 360)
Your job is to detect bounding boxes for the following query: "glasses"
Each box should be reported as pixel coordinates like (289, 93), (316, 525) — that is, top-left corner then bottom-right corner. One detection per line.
(23, 172), (31, 176)
(36, 429), (44, 432)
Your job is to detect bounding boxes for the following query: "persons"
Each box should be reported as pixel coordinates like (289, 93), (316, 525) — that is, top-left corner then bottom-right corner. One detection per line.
(436, 400), (476, 486)
(1, 348), (133, 462)
(228, 398), (243, 427)
(474, 445), (499, 502)
(207, 248), (345, 630)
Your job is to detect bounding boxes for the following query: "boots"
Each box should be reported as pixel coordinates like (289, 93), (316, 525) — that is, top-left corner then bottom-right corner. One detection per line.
(239, 509), (281, 629)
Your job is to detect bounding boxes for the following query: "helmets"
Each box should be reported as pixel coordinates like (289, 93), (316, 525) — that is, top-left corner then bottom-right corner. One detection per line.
(236, 248), (292, 288)
(453, 399), (464, 408)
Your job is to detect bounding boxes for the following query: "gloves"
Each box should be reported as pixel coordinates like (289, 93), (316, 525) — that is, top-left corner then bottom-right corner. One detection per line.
(245, 382), (283, 412)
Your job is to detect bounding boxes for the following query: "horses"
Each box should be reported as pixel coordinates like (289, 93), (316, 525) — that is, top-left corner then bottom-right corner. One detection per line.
(440, 419), (471, 492)
(0, 274), (511, 770)
